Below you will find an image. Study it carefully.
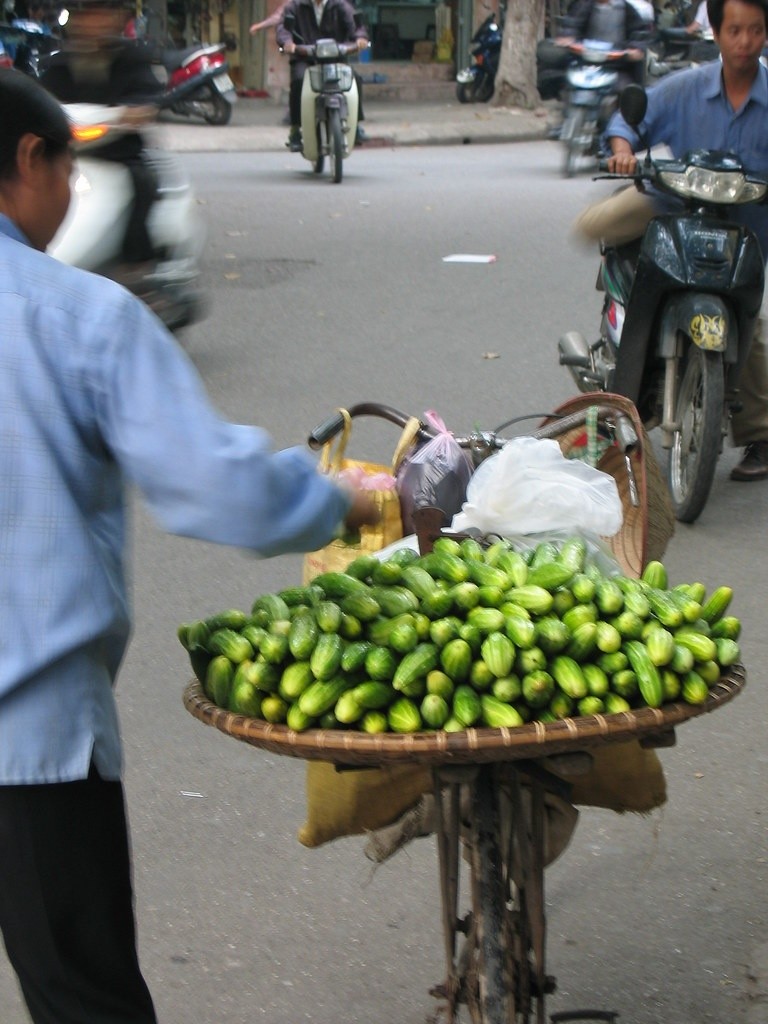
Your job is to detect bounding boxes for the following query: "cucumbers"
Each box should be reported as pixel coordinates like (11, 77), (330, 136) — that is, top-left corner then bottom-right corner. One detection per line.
(176, 536), (741, 732)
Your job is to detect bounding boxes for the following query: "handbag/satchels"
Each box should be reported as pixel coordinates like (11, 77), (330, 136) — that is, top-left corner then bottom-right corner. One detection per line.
(301, 407), (420, 588)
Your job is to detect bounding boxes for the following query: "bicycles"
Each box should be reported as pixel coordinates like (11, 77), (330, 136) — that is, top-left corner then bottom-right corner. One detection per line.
(310, 397), (638, 1024)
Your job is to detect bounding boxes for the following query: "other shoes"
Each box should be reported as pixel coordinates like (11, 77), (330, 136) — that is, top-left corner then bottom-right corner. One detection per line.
(355, 131), (363, 145)
(291, 134), (302, 152)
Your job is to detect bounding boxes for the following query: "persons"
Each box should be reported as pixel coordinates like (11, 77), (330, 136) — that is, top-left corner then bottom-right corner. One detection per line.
(248, 0), (292, 36)
(0, 61), (385, 1021)
(681, 1), (710, 33)
(547, 0), (652, 154)
(273, 0), (371, 154)
(595, 0), (768, 481)
(8, 1), (62, 43)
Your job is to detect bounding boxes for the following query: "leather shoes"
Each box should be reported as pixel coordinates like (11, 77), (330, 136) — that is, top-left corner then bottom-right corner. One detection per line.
(730, 444), (768, 479)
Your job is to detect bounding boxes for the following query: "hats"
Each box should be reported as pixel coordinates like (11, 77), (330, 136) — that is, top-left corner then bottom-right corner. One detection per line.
(534, 392), (676, 585)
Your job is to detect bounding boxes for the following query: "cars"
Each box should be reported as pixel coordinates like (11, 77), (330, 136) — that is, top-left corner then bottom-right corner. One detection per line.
(44, 121), (211, 336)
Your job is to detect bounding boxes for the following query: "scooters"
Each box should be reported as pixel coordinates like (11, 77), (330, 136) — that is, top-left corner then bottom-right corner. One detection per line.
(1, 1), (70, 116)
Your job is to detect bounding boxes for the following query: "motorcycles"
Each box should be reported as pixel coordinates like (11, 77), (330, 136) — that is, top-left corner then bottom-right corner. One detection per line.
(94, 20), (238, 125)
(553, 36), (669, 177)
(455, 12), (576, 104)
(274, 38), (363, 183)
(558, 84), (767, 525)
